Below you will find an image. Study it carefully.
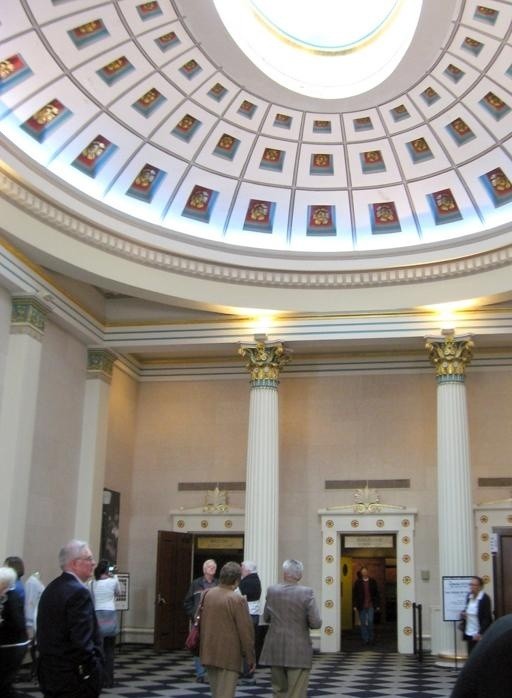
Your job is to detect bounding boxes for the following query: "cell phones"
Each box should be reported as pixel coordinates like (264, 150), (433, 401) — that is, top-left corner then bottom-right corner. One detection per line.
(109, 566), (115, 572)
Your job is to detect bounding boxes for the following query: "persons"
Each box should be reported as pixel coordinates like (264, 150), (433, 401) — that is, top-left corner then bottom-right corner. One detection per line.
(352, 567), (381, 646)
(258, 559), (322, 698)
(0, 542), (123, 697)
(183, 559), (261, 698)
(458, 577), (492, 653)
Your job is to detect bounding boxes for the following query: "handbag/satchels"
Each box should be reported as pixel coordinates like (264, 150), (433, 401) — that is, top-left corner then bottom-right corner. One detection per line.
(458, 620), (465, 631)
(185, 616), (199, 657)
(95, 610), (119, 636)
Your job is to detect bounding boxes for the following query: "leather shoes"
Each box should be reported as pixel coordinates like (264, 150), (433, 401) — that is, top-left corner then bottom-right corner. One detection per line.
(196, 677), (203, 683)
